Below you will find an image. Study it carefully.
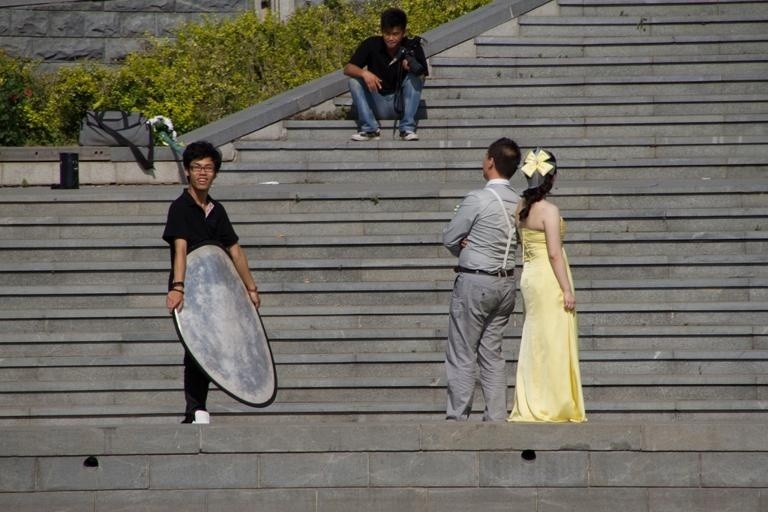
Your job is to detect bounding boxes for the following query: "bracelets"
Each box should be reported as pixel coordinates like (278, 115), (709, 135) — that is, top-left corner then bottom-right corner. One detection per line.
(172, 282), (184, 294)
(248, 286), (257, 292)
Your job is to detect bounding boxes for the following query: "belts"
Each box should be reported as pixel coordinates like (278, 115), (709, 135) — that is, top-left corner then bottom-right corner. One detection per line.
(455, 265), (514, 276)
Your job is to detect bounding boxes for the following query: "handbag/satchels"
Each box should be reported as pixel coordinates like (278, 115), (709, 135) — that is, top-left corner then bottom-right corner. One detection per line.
(79, 109), (153, 169)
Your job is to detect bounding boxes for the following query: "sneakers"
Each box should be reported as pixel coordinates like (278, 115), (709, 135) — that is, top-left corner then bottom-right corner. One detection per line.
(399, 131), (419, 141)
(352, 130), (381, 141)
(191, 411), (210, 424)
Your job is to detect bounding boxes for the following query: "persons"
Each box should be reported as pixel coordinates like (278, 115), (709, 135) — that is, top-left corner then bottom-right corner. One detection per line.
(515, 150), (587, 422)
(344, 8), (429, 141)
(162, 141), (260, 424)
(443, 138), (520, 422)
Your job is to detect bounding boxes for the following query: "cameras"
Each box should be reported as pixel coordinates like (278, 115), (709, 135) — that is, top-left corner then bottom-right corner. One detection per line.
(396, 48), (425, 76)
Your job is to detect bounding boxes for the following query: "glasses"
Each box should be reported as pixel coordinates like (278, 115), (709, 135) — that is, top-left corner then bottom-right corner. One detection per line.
(190, 165), (214, 171)
